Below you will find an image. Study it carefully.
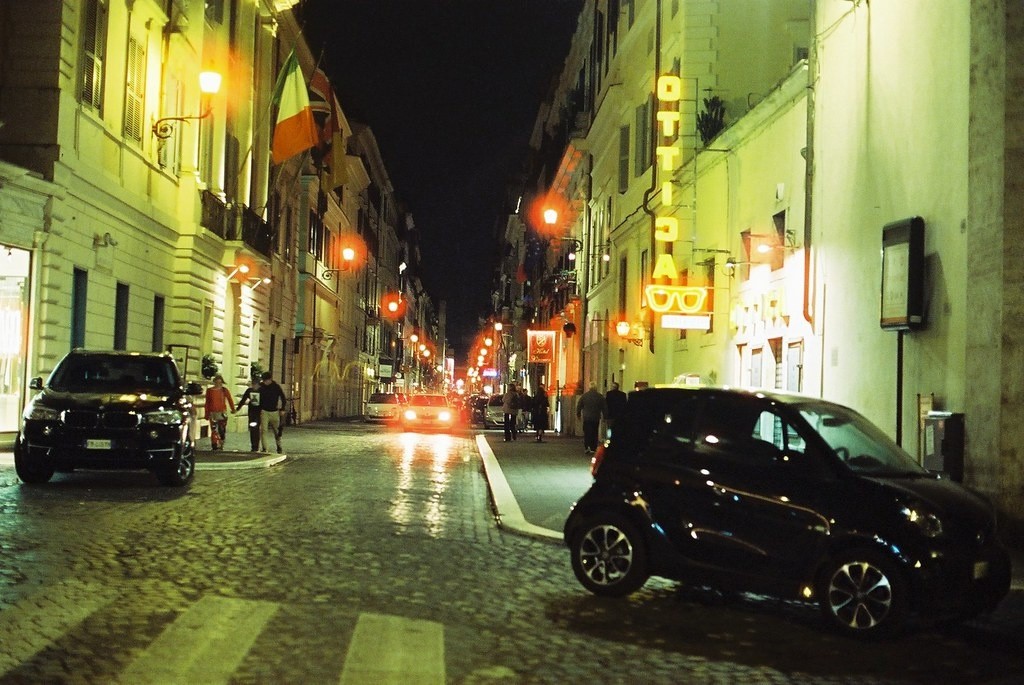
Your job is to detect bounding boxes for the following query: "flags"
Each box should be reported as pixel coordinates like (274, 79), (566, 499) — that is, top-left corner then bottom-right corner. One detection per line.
(273, 48), (320, 164)
(307, 67), (334, 174)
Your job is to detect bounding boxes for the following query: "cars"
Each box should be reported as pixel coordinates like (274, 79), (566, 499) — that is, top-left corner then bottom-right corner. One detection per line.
(561, 388), (1013, 675)
(363, 390), (526, 432)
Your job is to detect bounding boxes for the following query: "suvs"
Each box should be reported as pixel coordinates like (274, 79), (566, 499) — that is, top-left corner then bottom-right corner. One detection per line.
(13, 347), (204, 485)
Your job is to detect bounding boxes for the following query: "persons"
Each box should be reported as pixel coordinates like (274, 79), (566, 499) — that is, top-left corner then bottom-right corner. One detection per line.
(606, 382), (627, 427)
(516, 386), (532, 432)
(235, 378), (262, 451)
(502, 384), (520, 441)
(205, 375), (236, 449)
(577, 381), (608, 453)
(532, 387), (549, 440)
(257, 372), (286, 454)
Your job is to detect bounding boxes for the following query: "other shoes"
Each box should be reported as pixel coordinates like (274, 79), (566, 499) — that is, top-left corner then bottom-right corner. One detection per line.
(277, 448), (282, 453)
(590, 448), (597, 452)
(537, 435), (542, 441)
(585, 450), (590, 454)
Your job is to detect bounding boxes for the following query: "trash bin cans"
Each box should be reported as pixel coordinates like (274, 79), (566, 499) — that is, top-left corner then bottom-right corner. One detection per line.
(924, 410), (965, 472)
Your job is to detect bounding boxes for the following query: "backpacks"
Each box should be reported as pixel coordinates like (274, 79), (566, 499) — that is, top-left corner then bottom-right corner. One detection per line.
(507, 392), (520, 410)
